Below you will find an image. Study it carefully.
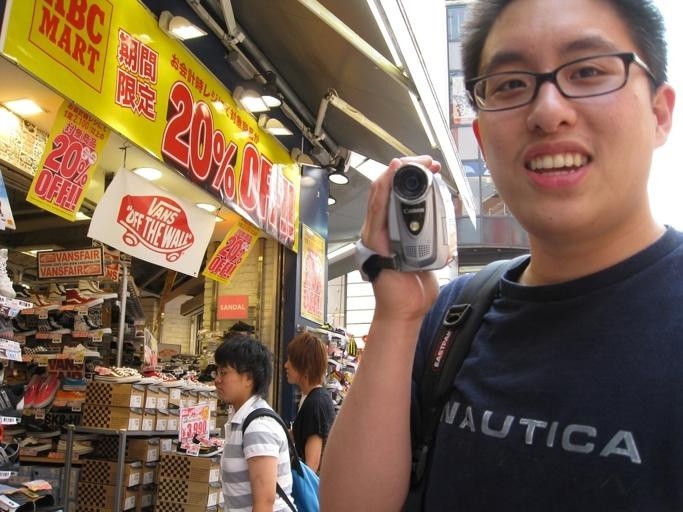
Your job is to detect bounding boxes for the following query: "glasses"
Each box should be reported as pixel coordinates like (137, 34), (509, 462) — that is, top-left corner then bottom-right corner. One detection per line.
(464, 50), (658, 112)
(215, 369), (240, 377)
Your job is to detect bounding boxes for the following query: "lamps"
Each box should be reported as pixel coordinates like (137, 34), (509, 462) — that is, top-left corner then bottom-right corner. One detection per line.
(159, 11), (350, 186)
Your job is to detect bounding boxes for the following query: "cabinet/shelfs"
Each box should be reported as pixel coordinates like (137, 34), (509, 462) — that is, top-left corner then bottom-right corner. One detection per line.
(58, 366), (235, 511)
(308, 326), (366, 408)
(0, 248), (146, 512)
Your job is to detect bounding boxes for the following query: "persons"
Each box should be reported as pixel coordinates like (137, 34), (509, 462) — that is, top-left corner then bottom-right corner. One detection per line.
(214, 337), (298, 512)
(318, 1), (683, 512)
(284, 333), (336, 474)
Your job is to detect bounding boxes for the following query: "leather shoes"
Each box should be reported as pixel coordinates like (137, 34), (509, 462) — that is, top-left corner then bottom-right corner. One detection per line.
(230, 320), (255, 332)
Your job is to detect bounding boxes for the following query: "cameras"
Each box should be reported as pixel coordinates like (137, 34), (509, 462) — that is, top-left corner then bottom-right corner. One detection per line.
(386, 163), (460, 273)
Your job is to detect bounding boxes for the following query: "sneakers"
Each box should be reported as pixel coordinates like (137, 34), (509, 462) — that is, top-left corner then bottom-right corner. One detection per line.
(24, 345), (59, 361)
(327, 355), (357, 391)
(0, 441), (20, 466)
(0, 369), (88, 410)
(317, 322), (345, 338)
(1, 247), (17, 297)
(95, 366), (215, 392)
(1, 314), (112, 339)
(63, 345), (101, 359)
(4, 419), (94, 455)
(177, 437), (225, 453)
(12, 281), (118, 308)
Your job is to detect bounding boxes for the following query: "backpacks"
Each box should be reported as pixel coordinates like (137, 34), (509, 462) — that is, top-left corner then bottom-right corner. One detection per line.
(244, 408), (319, 512)
